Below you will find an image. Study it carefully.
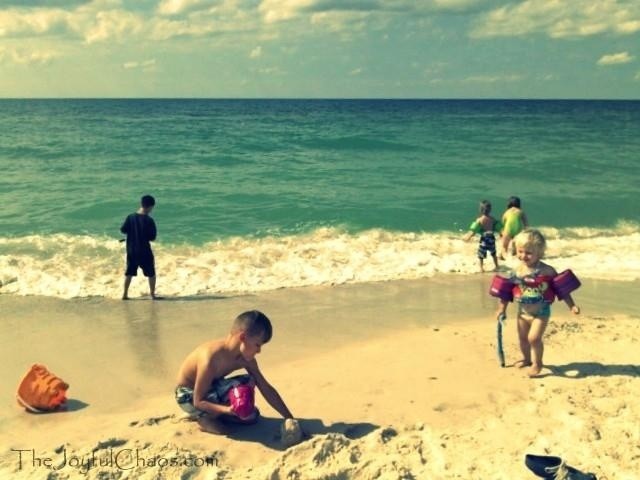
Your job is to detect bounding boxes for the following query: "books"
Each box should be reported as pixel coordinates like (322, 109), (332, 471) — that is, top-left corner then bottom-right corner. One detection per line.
(228, 385), (256, 419)
(13, 363), (71, 413)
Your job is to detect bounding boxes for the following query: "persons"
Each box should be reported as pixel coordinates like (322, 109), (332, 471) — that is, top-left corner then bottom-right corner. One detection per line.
(498, 194), (530, 258)
(171, 307), (310, 439)
(116, 193), (161, 301)
(492, 227), (581, 378)
(460, 199), (503, 274)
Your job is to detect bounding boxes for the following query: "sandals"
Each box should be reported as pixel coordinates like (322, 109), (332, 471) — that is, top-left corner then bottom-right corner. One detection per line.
(525, 454), (597, 480)
